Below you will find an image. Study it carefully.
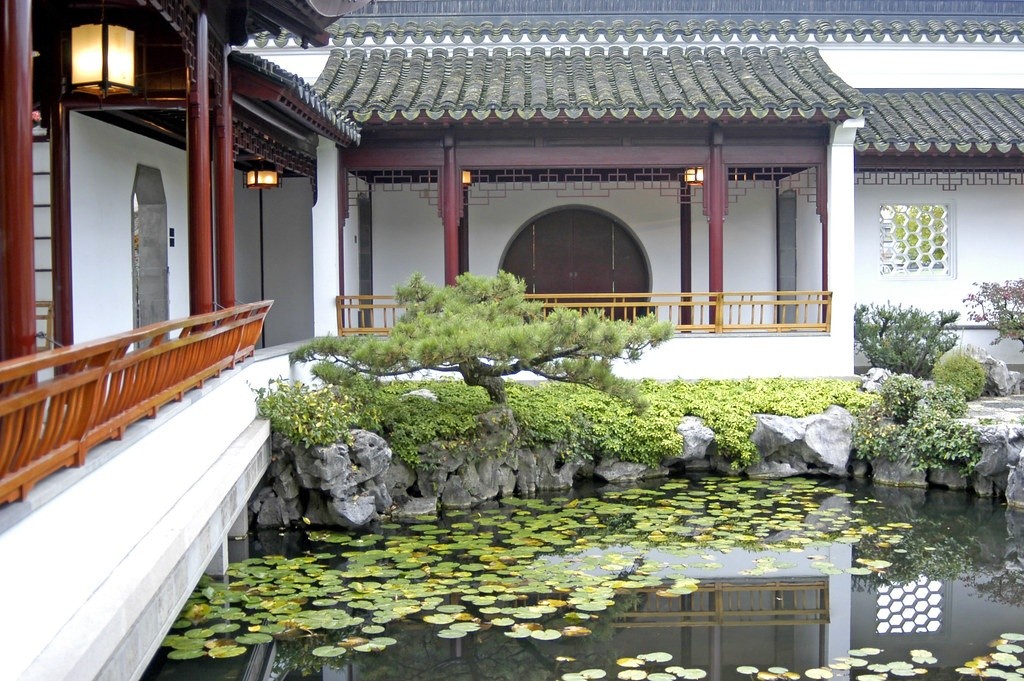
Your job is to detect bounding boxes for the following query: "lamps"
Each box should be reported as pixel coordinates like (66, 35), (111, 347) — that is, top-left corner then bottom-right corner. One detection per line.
(243, 162), (283, 191)
(62, 0), (146, 98)
(462, 171), (473, 187)
(684, 167), (704, 186)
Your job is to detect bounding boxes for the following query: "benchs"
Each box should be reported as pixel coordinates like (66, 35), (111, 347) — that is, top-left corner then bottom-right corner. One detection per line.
(1, 300), (274, 509)
